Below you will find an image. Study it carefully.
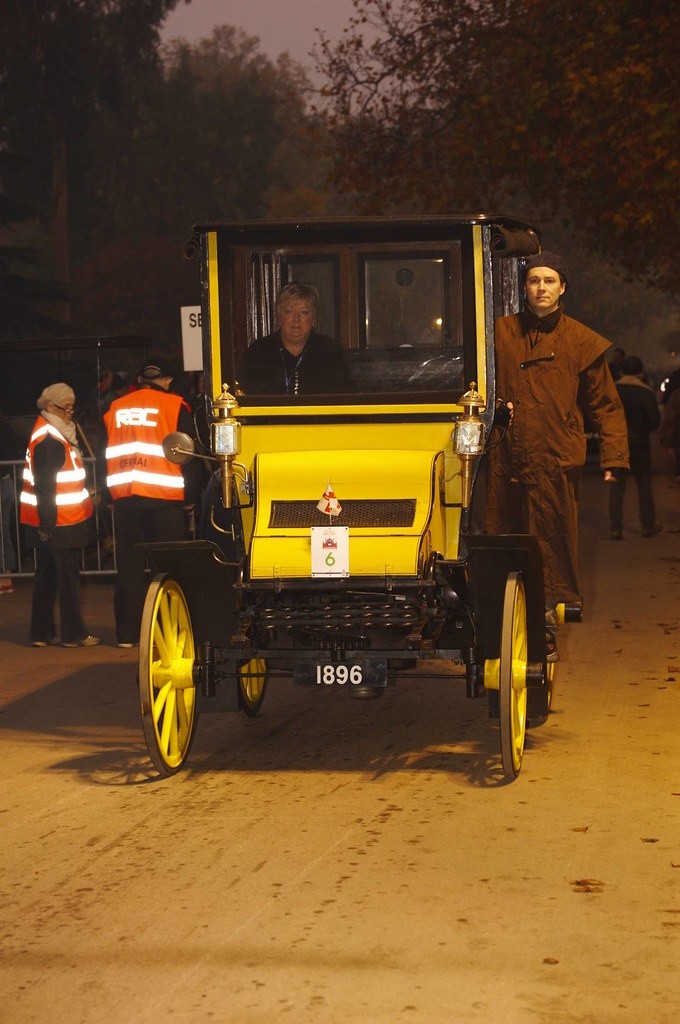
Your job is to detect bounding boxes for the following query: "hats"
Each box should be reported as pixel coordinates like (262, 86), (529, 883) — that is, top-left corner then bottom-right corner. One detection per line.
(139, 358), (172, 379)
(37, 383), (74, 410)
(623, 356), (642, 374)
(522, 250), (569, 296)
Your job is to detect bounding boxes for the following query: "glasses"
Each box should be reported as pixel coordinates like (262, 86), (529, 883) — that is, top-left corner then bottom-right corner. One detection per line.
(55, 404), (77, 412)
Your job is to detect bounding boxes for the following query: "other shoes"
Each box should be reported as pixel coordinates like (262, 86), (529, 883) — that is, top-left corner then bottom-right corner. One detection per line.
(545, 630), (560, 663)
(63, 634), (101, 647)
(117, 642), (137, 648)
(642, 523), (663, 538)
(32, 640), (48, 647)
(611, 531), (622, 540)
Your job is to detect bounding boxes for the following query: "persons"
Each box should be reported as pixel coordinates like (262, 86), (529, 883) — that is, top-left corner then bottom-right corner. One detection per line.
(236, 282), (359, 396)
(661, 368), (680, 484)
(20, 383), (101, 647)
(77, 359), (213, 647)
(610, 355), (663, 539)
(0, 421), (20, 594)
(479, 252), (629, 663)
(608, 348), (625, 382)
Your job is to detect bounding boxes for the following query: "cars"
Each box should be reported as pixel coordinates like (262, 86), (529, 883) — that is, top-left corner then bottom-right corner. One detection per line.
(134, 210), (583, 782)
